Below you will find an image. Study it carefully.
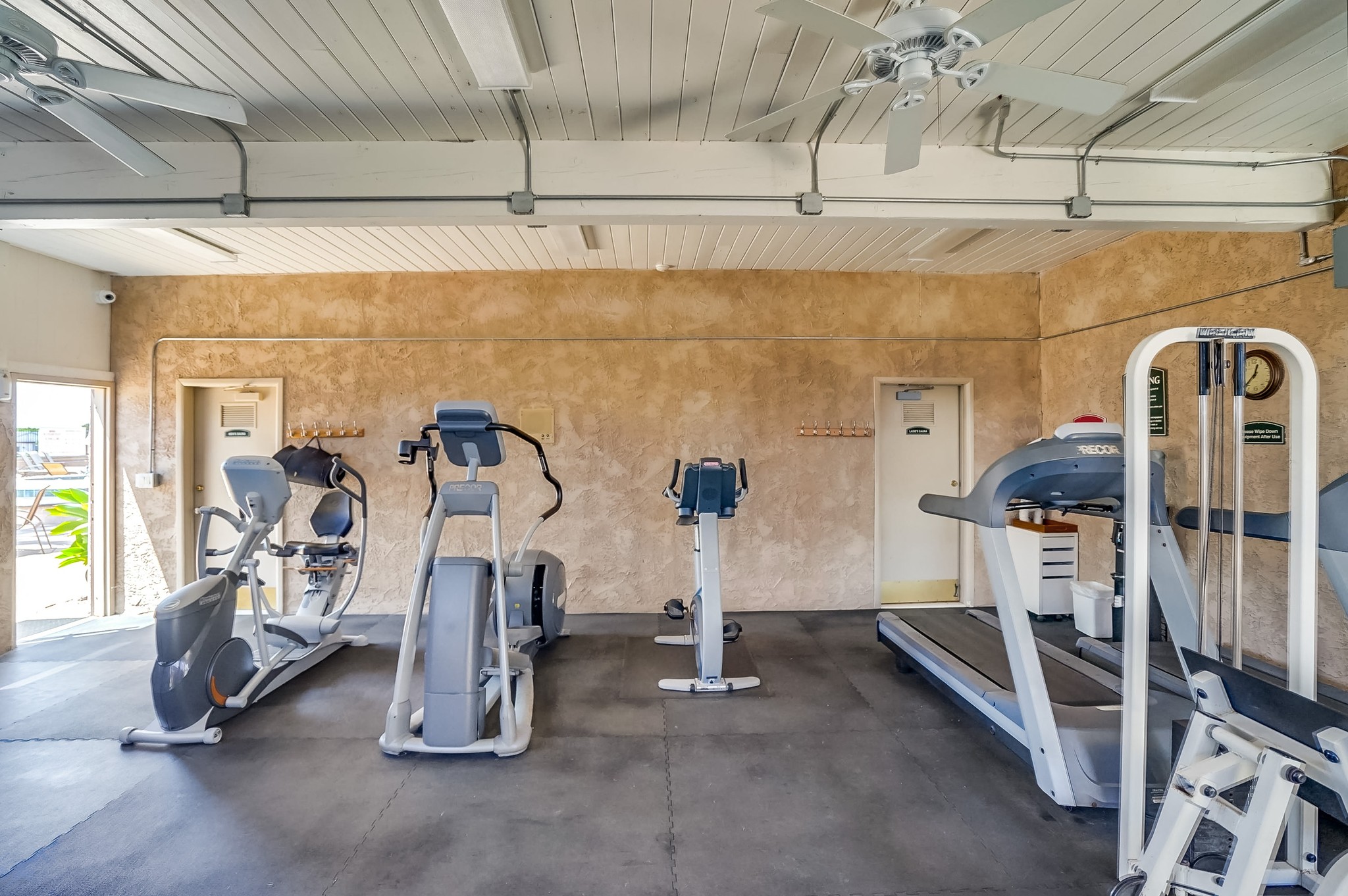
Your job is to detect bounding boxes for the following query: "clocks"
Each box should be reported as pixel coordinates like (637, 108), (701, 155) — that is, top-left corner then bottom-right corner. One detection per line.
(1232, 349), (1286, 400)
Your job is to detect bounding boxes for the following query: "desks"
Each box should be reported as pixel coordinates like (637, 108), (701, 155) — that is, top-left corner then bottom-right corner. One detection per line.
(1006, 525), (1078, 621)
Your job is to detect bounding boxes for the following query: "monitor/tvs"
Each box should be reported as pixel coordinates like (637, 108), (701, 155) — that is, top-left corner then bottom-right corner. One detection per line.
(224, 455), (292, 523)
(682, 462), (737, 516)
(434, 401), (507, 466)
(1011, 422), (1125, 506)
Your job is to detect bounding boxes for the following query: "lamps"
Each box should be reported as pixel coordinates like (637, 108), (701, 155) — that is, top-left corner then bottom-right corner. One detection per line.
(1149, 0), (1348, 103)
(438, 0), (532, 91)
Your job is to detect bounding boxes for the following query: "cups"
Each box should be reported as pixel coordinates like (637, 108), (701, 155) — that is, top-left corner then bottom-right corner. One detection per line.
(1019, 510), (1031, 522)
(1034, 510), (1043, 525)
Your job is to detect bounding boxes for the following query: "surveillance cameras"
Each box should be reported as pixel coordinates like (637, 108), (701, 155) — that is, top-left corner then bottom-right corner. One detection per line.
(95, 289), (116, 304)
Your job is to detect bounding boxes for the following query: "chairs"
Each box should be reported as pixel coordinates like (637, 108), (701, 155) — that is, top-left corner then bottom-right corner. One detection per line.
(16, 485), (54, 553)
(17, 451), (79, 476)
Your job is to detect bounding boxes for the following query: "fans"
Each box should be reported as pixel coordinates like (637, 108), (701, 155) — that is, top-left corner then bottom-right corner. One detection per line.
(724, 0), (1129, 178)
(0, 5), (247, 179)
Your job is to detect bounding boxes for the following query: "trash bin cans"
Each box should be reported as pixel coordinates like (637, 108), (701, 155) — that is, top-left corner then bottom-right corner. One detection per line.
(1070, 580), (1113, 638)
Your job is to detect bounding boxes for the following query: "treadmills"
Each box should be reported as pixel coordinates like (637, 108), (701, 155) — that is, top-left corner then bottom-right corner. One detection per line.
(875, 420), (1225, 813)
(1075, 473), (1348, 826)
